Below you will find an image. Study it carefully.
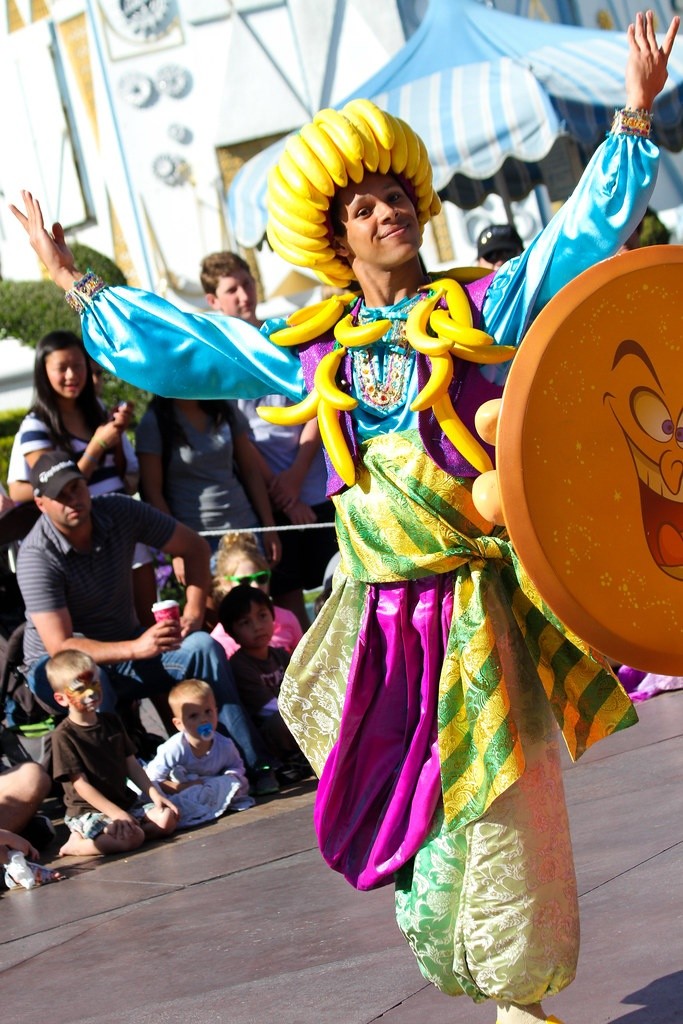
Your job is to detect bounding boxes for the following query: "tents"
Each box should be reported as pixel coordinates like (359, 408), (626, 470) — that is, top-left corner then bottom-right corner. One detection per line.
(226, 0), (682, 250)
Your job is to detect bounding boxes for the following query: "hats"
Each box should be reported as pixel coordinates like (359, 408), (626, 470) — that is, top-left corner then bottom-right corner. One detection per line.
(27, 450), (90, 499)
(268, 95), (442, 288)
(476, 223), (523, 260)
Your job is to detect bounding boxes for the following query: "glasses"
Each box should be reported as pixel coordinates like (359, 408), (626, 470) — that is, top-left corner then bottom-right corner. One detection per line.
(223, 570), (271, 586)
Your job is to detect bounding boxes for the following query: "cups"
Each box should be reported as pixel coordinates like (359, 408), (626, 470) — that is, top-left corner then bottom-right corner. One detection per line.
(152, 599), (181, 637)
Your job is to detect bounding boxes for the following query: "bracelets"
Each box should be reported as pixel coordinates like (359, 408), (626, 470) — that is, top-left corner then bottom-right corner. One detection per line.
(83, 453), (98, 464)
(92, 434), (109, 450)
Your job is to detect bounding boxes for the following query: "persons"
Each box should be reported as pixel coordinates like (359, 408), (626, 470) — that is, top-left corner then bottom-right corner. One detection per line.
(476, 224), (524, 271)
(126, 678), (256, 828)
(1, 251), (341, 863)
(16, 452), (280, 795)
(8, 7), (682, 1024)
(44, 649), (179, 857)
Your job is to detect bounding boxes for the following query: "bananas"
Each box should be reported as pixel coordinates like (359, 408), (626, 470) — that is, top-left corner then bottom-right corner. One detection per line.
(265, 98), (443, 287)
(255, 266), (516, 488)
